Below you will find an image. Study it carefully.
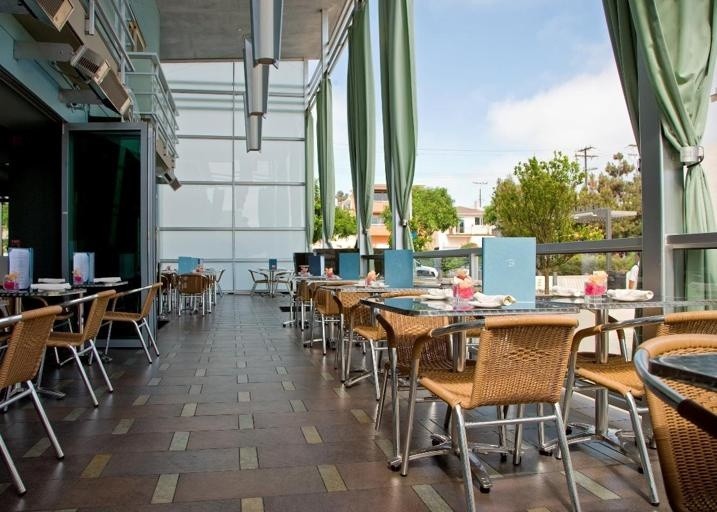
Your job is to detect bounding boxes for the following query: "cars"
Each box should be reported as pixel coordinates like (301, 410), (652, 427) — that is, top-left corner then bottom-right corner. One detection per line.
(415, 267), (438, 281)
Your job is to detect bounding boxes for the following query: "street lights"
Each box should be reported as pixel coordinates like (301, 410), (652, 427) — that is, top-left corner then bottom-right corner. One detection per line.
(473, 180), (489, 208)
(571, 207), (639, 271)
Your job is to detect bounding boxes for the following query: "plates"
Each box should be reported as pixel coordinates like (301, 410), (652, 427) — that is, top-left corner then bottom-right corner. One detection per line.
(420, 301), (446, 306)
(610, 296), (638, 302)
(354, 284), (365, 288)
(420, 294), (446, 300)
(466, 301), (501, 308)
(370, 285), (388, 288)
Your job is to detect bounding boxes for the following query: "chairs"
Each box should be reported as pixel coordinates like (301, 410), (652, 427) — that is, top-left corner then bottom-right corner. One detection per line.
(0, 306), (65, 495)
(631, 334), (716, 511)
(557, 307), (717, 506)
(0, 253), (164, 411)
(401, 313), (583, 512)
(281, 263), (630, 494)
(160, 265), (224, 317)
(248, 267), (295, 299)
